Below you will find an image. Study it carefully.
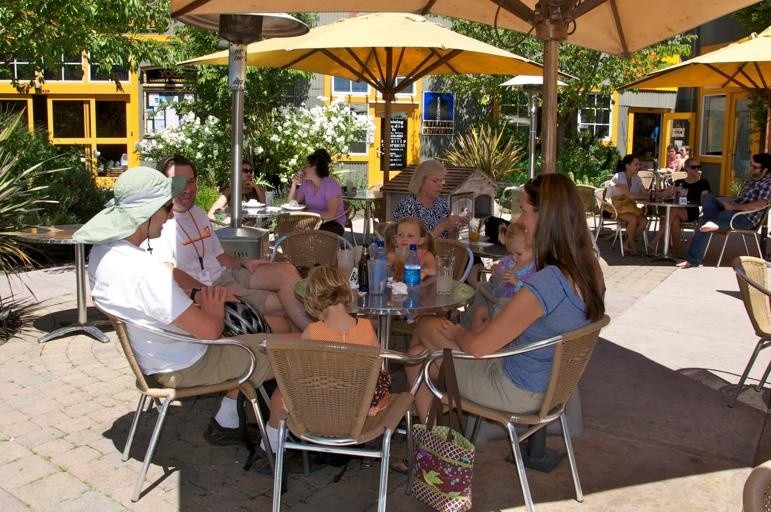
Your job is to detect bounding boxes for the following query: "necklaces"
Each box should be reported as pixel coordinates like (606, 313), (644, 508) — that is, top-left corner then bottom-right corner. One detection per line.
(174, 209), (187, 213)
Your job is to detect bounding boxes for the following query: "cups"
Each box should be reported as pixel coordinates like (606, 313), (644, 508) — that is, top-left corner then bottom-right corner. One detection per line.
(403, 244), (421, 299)
(265, 191), (274, 207)
(351, 246), (362, 265)
(468, 218), (480, 242)
(432, 254), (457, 296)
(367, 294), (388, 309)
(336, 249), (354, 274)
(296, 171), (304, 185)
(367, 260), (388, 295)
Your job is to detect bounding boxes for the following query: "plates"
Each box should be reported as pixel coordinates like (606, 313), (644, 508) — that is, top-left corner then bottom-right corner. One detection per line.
(244, 203), (265, 208)
(282, 203), (307, 211)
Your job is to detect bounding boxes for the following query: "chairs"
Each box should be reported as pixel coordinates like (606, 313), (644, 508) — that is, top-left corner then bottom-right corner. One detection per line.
(259, 339), (432, 511)
(742, 460), (770, 511)
(423, 315), (611, 510)
(93, 304), (287, 502)
(93, 170), (709, 352)
(701, 201), (770, 268)
(728, 255), (771, 408)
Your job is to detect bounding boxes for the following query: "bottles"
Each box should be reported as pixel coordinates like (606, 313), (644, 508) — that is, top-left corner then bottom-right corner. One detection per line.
(370, 239), (386, 259)
(358, 243), (371, 290)
(403, 299), (420, 309)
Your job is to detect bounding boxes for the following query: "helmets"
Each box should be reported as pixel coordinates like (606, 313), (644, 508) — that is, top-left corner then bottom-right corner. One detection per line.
(219, 295), (272, 338)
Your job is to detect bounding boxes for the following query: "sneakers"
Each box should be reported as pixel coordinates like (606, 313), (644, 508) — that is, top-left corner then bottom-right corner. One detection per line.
(674, 260), (705, 268)
(203, 418), (262, 448)
(698, 220), (720, 234)
(253, 446), (294, 472)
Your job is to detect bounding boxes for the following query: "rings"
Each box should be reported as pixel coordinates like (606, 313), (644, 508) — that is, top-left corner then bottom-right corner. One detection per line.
(456, 221), (458, 224)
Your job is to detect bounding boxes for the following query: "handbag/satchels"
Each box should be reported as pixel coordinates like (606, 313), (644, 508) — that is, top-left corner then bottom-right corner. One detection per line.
(409, 424), (476, 511)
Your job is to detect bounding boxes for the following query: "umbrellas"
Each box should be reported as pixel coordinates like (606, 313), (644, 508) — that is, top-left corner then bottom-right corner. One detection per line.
(613, 25), (771, 91)
(173, 13), (579, 185)
(172, 0), (765, 173)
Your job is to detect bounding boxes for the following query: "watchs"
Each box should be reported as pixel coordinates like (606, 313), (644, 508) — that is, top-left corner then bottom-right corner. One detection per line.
(190, 287), (202, 304)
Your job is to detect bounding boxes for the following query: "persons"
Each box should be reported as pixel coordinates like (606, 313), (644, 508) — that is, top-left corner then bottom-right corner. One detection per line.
(299, 264), (409, 473)
(207, 160), (266, 220)
(287, 150), (347, 237)
(385, 216), (435, 279)
(464, 224), (538, 329)
(391, 172), (605, 476)
(665, 145), (689, 171)
(602, 154), (671, 257)
(391, 159), (486, 288)
(649, 157), (710, 257)
(71, 166), (304, 474)
(677, 153), (770, 267)
(141, 155), (316, 335)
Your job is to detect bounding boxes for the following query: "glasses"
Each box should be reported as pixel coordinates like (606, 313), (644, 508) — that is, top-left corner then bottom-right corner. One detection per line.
(750, 162), (762, 169)
(242, 168), (253, 175)
(688, 164), (701, 170)
(160, 203), (176, 211)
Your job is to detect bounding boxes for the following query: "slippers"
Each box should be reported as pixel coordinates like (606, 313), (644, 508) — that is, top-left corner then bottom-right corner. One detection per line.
(390, 457), (411, 476)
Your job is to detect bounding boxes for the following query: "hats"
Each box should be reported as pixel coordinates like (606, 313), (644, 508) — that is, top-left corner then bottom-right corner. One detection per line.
(74, 167), (187, 245)
